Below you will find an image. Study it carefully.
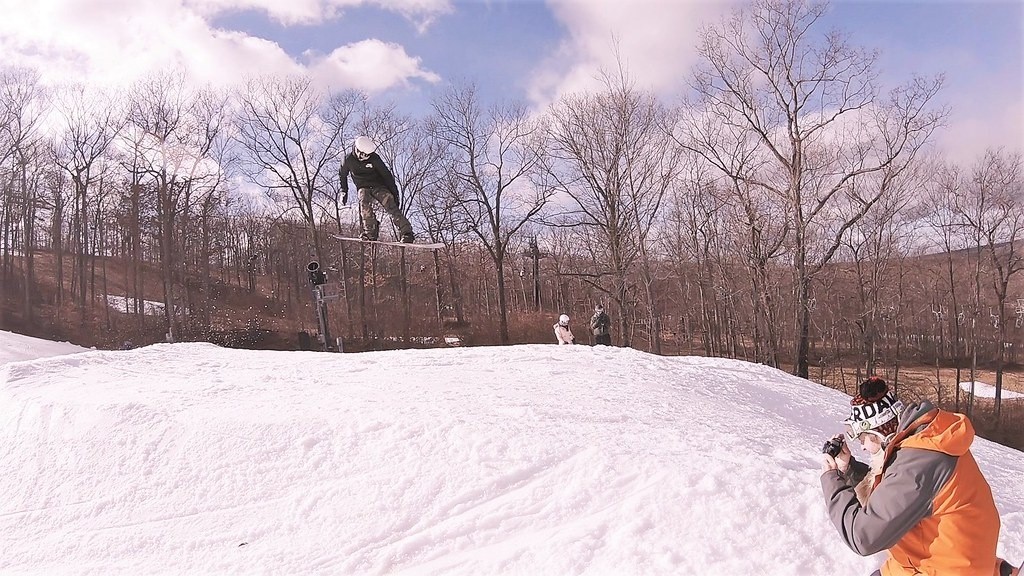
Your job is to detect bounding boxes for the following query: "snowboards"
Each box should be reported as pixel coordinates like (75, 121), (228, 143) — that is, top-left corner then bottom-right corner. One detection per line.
(332, 233), (446, 249)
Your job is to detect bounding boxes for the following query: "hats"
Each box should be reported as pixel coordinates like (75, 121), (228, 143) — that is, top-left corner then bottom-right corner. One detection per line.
(849, 377), (900, 440)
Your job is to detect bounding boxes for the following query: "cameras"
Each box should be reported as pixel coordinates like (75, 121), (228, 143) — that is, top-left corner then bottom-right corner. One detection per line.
(822, 438), (844, 457)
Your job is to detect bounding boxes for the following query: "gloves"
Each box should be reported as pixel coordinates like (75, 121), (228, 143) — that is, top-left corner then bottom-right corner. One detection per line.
(340, 193), (347, 206)
(391, 188), (400, 200)
(598, 317), (607, 326)
(572, 338), (576, 344)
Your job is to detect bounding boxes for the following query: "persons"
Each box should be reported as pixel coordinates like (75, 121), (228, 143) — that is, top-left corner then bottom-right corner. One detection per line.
(590, 305), (611, 346)
(553, 314), (576, 345)
(339, 136), (415, 243)
(820, 377), (1019, 576)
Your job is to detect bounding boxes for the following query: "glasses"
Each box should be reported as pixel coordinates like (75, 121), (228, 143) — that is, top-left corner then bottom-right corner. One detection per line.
(843, 418), (860, 441)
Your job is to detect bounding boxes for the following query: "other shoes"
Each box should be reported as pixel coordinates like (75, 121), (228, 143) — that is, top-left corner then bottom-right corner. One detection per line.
(360, 232), (376, 241)
(401, 232), (415, 243)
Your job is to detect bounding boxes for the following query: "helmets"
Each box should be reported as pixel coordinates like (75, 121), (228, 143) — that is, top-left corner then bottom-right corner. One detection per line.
(355, 136), (377, 158)
(560, 315), (569, 324)
(595, 305), (603, 312)
